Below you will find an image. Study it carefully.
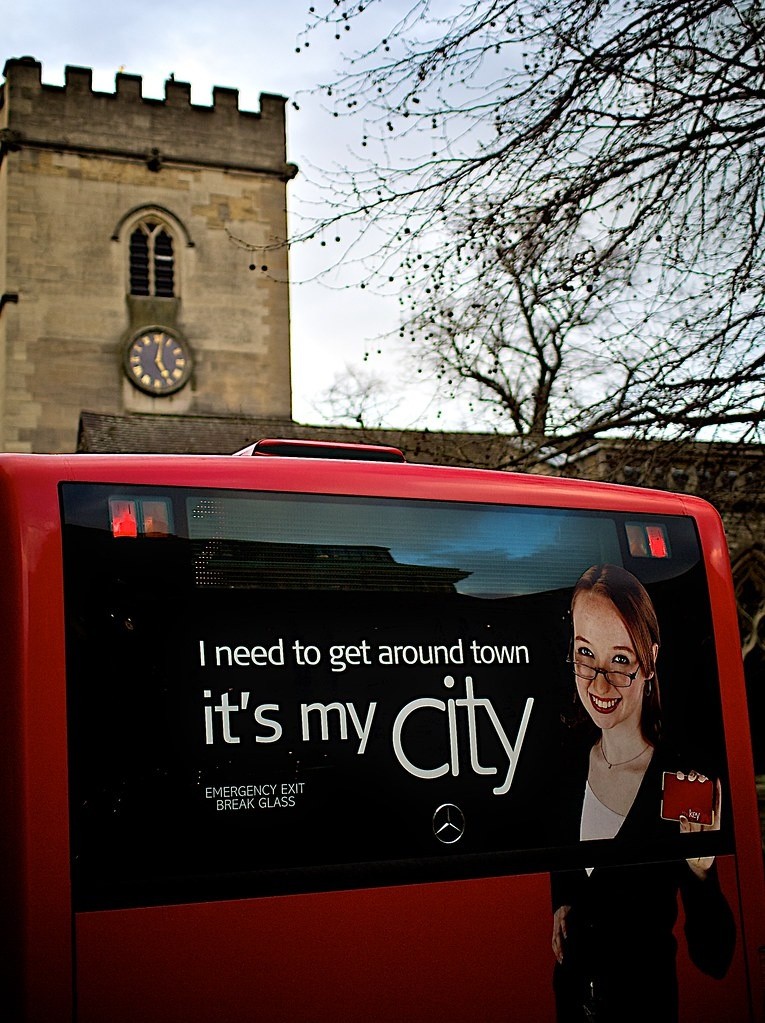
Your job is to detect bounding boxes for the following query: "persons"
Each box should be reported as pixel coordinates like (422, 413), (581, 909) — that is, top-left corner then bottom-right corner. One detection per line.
(542, 564), (735, 1023)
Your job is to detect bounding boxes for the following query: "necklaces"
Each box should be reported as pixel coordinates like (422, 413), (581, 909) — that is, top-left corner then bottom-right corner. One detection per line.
(600, 740), (650, 769)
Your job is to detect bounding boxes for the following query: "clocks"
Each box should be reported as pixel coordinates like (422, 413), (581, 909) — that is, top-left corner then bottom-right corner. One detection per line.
(122, 324), (194, 398)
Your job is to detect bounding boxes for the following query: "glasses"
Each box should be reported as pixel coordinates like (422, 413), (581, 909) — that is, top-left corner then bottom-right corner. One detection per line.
(566, 638), (642, 688)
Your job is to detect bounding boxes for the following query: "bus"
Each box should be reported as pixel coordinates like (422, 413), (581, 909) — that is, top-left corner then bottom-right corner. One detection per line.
(1, 437), (765, 1023)
